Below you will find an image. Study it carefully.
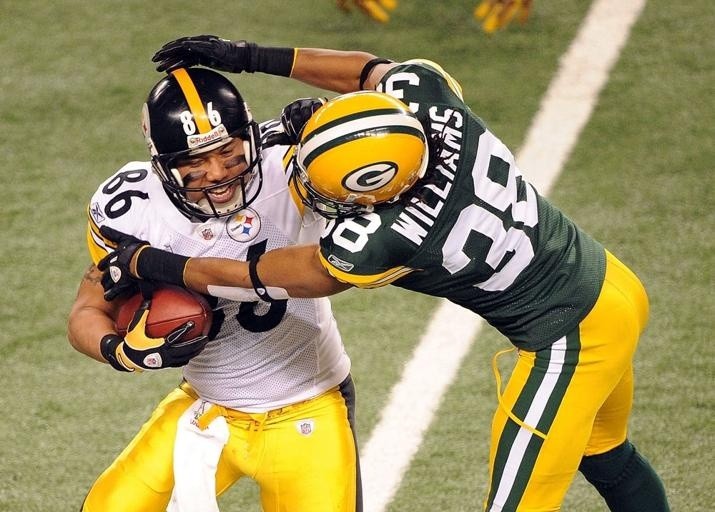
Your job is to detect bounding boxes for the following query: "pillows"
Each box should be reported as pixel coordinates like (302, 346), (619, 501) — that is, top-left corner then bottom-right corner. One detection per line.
(140, 68), (263, 218)
(293, 91), (430, 218)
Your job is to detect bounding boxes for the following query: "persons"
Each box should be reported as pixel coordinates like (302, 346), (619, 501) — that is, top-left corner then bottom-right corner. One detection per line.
(96, 33), (671, 512)
(337, 0), (532, 30)
(66, 65), (365, 512)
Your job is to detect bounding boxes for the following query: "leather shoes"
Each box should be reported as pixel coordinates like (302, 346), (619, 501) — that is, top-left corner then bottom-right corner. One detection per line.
(151, 34), (249, 73)
(282, 97), (325, 136)
(98, 224), (151, 302)
(107, 305), (207, 372)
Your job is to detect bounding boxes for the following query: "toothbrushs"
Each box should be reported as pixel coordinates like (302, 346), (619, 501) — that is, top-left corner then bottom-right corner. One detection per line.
(115, 283), (214, 345)
